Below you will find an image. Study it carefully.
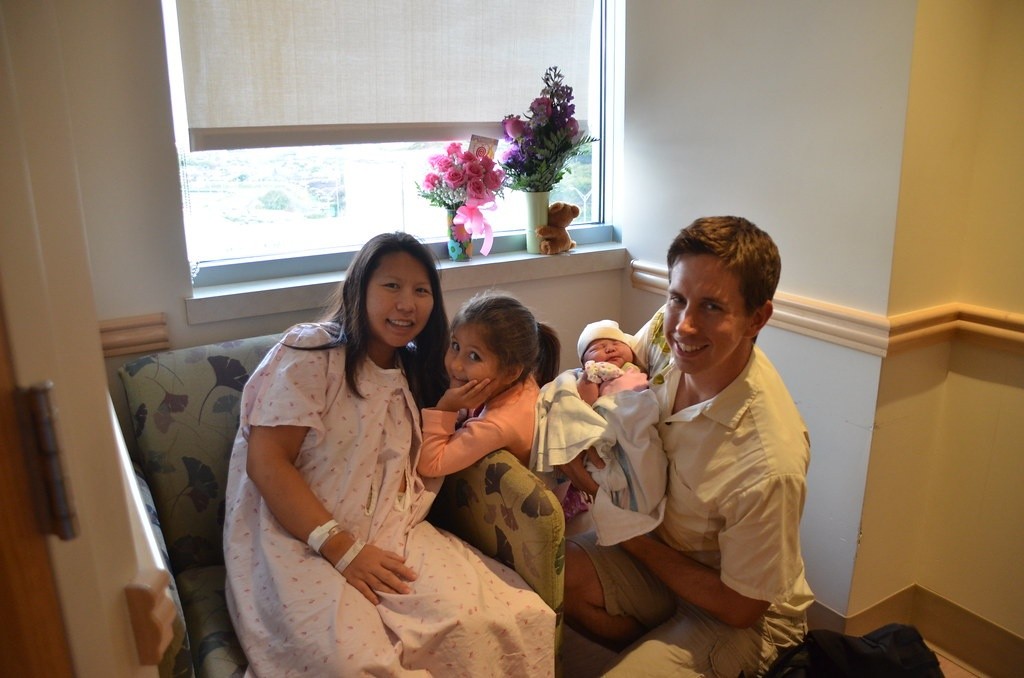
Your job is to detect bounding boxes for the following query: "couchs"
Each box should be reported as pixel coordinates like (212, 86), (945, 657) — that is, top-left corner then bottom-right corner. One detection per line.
(119, 334), (567, 678)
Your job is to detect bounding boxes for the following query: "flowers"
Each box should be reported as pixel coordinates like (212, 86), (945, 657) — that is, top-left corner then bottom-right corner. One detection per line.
(415, 140), (507, 257)
(499, 66), (600, 197)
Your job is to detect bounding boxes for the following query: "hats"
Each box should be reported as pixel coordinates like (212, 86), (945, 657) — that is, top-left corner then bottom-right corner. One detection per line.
(577, 320), (637, 367)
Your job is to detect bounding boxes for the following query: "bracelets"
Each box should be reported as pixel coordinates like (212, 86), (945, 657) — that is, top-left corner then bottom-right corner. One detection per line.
(309, 520), (344, 554)
(335, 540), (365, 573)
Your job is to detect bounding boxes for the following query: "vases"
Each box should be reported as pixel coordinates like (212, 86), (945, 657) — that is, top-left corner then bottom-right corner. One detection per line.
(446, 210), (472, 262)
(521, 192), (579, 254)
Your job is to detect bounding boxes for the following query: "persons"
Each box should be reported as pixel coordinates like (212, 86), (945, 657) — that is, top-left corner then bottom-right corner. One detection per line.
(576, 320), (666, 515)
(224, 232), (556, 678)
(561, 216), (816, 678)
(418, 295), (594, 536)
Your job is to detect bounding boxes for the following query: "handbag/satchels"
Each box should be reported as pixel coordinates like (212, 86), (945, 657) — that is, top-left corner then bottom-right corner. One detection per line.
(760, 623), (944, 678)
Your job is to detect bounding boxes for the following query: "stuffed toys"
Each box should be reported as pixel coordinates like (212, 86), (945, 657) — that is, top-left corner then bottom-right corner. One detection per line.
(536, 202), (579, 255)
